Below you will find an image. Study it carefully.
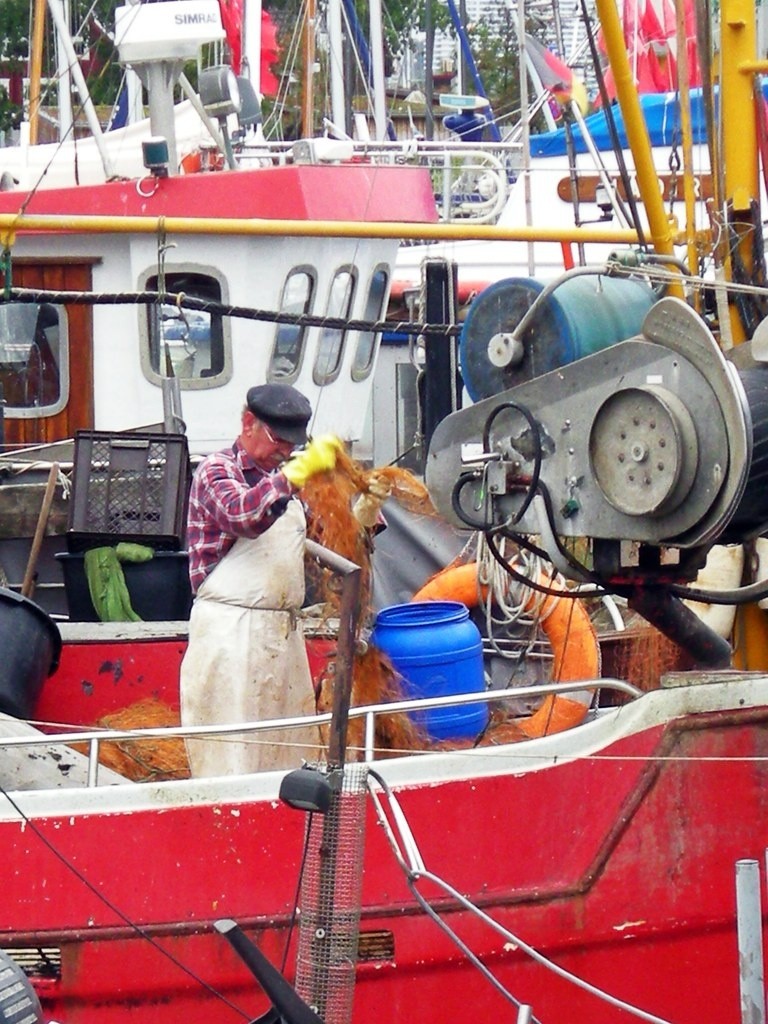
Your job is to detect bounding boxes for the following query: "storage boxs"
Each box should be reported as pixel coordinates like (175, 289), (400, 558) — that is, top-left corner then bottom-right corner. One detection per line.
(69, 428), (192, 550)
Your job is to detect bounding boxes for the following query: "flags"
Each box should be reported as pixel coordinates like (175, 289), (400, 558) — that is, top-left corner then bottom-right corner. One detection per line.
(524, 33), (587, 114)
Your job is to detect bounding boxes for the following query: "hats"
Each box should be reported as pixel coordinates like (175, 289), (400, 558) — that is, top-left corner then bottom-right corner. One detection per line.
(246, 382), (313, 443)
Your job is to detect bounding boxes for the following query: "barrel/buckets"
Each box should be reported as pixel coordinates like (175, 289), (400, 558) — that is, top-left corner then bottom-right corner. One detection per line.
(0, 586), (63, 721)
(370, 600), (489, 742)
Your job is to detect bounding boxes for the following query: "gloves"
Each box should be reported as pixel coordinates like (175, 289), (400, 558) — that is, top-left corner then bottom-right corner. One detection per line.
(280, 435), (349, 489)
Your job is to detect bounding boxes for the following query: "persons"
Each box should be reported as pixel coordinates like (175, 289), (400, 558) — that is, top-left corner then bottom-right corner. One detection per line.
(179, 381), (394, 778)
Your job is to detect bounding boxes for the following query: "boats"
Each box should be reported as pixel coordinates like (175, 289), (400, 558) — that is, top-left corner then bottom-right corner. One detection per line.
(0, 0), (768, 1024)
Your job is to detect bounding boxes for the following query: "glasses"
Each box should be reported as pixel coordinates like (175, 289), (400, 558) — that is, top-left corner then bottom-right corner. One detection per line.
(263, 426), (306, 450)
